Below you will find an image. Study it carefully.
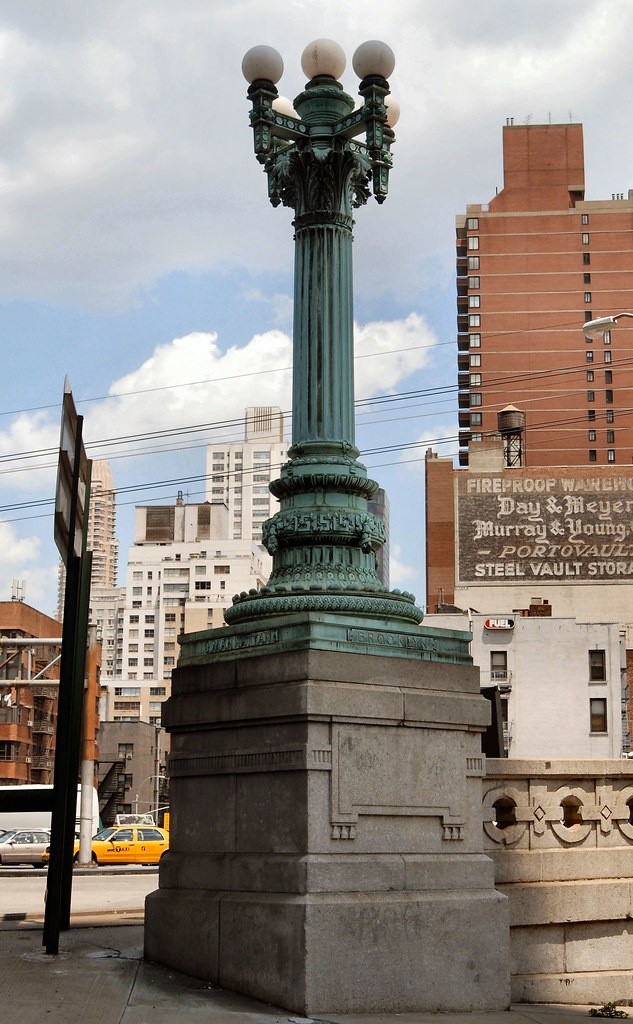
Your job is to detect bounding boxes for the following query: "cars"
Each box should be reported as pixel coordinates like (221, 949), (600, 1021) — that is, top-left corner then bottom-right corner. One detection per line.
(40, 814), (169, 866)
(0, 828), (51, 868)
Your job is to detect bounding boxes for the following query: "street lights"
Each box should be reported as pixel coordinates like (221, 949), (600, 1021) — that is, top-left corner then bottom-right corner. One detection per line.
(135, 774), (169, 812)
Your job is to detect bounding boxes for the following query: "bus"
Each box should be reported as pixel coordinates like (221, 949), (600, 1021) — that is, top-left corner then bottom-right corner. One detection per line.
(0, 785), (100, 840)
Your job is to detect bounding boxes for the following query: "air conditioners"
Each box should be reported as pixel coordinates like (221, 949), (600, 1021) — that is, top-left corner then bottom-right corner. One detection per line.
(25, 757), (31, 764)
(27, 721), (33, 727)
